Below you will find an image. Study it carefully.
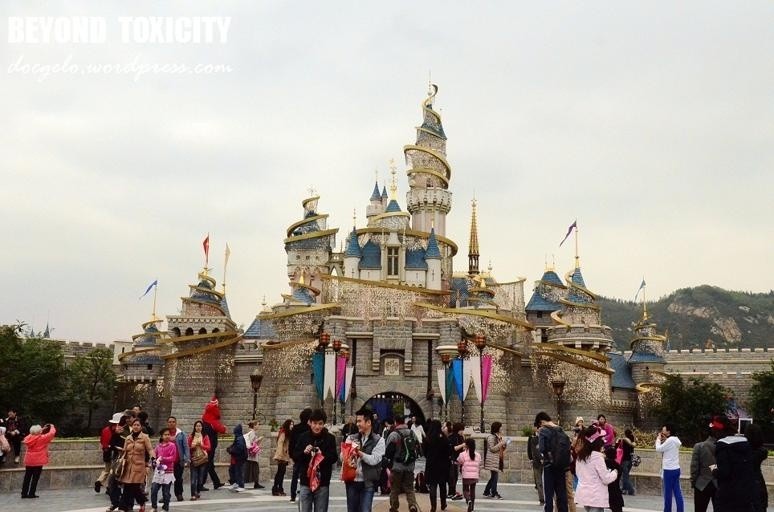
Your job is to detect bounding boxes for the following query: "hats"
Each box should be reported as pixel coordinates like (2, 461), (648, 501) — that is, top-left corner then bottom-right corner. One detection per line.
(210, 396), (218, 405)
(108, 410), (135, 424)
(584, 427), (607, 443)
(575, 416), (584, 426)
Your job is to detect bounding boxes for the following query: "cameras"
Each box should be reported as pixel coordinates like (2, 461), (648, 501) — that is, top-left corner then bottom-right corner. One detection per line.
(46, 424), (50, 427)
(311, 447), (318, 452)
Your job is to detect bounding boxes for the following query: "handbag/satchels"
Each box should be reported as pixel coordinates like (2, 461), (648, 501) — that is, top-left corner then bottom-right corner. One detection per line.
(629, 452), (642, 466)
(109, 449), (127, 476)
(248, 441), (263, 457)
(614, 438), (625, 465)
(308, 453), (325, 492)
(341, 442), (358, 481)
(191, 442), (209, 467)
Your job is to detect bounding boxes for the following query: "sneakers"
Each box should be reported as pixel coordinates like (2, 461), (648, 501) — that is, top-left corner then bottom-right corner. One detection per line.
(94, 480), (101, 492)
(235, 487), (245, 492)
(150, 508), (157, 512)
(214, 482), (226, 489)
(448, 491), (463, 500)
(159, 497), (164, 504)
(620, 487), (627, 494)
(176, 495), (184, 501)
(409, 506), (417, 511)
(199, 487), (209, 491)
(160, 509), (167, 512)
(139, 504), (146, 512)
(105, 506), (125, 512)
(254, 483), (265, 489)
(483, 494), (492, 498)
(190, 494), (200, 500)
(491, 494), (503, 500)
(466, 500), (472, 512)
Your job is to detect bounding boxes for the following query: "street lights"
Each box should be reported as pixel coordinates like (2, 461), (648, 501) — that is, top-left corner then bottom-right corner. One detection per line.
(249, 367), (263, 427)
(458, 340), (468, 427)
(330, 337), (342, 425)
(550, 380), (567, 426)
(474, 333), (488, 433)
(440, 352), (451, 422)
(341, 349), (350, 425)
(317, 331), (329, 410)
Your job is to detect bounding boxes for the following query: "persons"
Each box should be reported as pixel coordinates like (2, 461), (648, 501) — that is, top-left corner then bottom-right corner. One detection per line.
(710, 413), (757, 512)
(742, 424), (768, 511)
(691, 417), (725, 511)
(651, 420), (684, 511)
(0, 419), (11, 462)
(19, 422), (57, 498)
(2, 408), (26, 464)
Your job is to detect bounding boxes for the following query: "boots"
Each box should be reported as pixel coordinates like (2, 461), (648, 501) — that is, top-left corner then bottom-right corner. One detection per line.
(272, 486), (286, 496)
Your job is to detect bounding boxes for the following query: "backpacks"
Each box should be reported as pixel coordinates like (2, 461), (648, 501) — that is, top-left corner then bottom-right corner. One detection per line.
(544, 423), (570, 467)
(415, 470), (430, 493)
(394, 429), (417, 464)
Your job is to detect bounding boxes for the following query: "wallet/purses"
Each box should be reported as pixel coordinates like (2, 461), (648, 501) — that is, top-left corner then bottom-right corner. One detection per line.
(310, 446), (318, 456)
